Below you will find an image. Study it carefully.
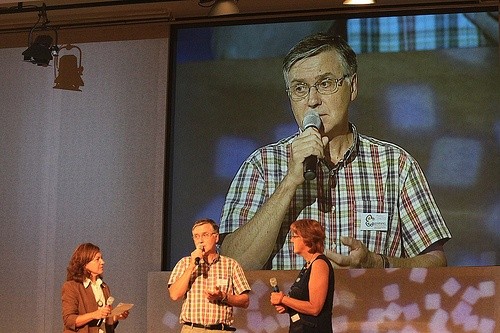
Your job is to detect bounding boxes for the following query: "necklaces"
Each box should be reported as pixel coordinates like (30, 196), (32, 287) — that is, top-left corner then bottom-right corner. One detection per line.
(95, 295), (104, 307)
(307, 253), (314, 264)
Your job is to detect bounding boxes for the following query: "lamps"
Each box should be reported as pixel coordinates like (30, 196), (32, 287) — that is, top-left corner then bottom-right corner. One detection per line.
(198, 0), (240, 16)
(18, 2), (59, 67)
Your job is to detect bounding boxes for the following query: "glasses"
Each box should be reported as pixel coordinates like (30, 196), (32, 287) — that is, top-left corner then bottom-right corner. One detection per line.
(286, 74), (348, 99)
(192, 233), (215, 240)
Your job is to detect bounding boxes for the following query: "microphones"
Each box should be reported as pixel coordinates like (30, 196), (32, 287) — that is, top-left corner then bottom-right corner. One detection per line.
(299, 110), (321, 180)
(195, 244), (204, 265)
(96, 296), (115, 327)
(270, 276), (280, 293)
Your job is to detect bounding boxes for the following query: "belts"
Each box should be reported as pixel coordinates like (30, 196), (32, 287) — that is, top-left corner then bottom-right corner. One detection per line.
(184, 322), (236, 331)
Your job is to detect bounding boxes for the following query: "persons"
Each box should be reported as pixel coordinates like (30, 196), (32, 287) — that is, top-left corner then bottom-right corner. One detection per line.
(217, 32), (456, 270)
(167, 218), (251, 333)
(61, 244), (129, 333)
(270, 220), (335, 333)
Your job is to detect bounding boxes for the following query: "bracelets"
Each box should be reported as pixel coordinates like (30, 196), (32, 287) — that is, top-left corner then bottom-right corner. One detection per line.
(279, 294), (286, 303)
(378, 253), (390, 268)
(221, 294), (228, 302)
